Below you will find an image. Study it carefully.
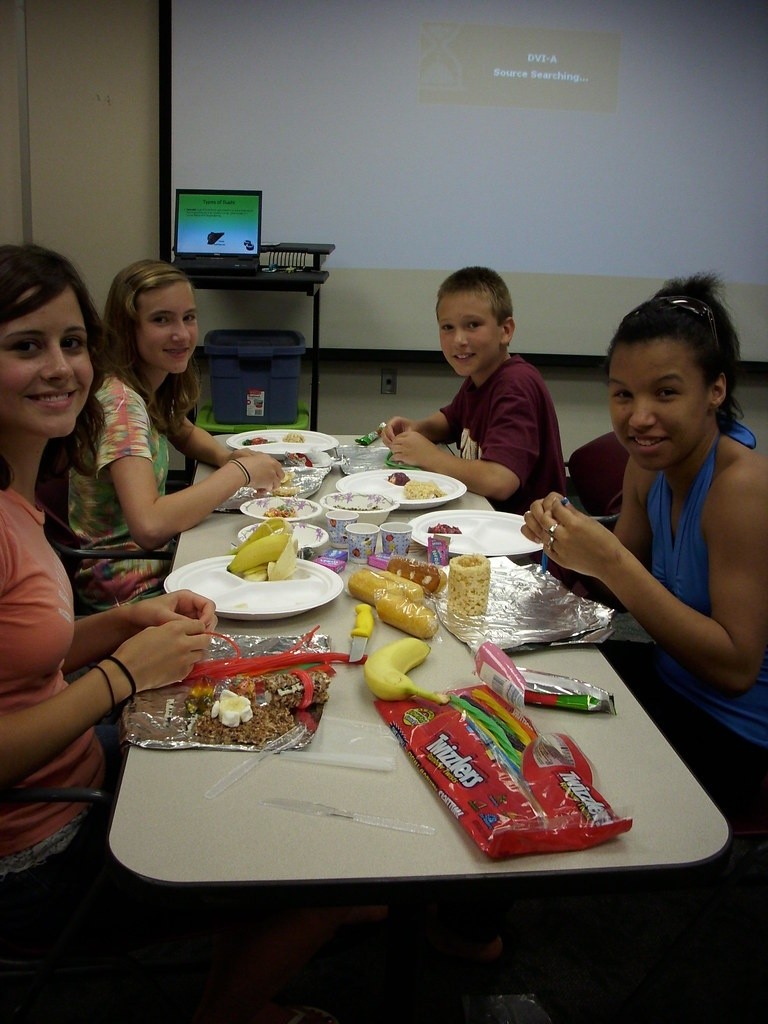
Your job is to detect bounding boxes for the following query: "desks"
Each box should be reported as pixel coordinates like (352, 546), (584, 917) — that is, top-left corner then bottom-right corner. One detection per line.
(106, 432), (732, 1024)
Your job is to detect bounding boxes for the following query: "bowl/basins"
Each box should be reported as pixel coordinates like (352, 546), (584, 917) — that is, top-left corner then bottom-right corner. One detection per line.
(320, 491), (400, 526)
(240, 496), (323, 524)
(237, 522), (329, 556)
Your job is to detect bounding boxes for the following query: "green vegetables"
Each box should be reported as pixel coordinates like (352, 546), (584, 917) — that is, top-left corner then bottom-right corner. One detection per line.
(242, 439), (252, 445)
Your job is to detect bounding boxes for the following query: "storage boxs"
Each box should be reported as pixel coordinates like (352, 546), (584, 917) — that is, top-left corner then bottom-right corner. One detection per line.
(204, 329), (306, 426)
(369, 553), (391, 570)
(428, 534), (453, 566)
(195, 398), (308, 436)
(313, 556), (346, 573)
(318, 548), (348, 562)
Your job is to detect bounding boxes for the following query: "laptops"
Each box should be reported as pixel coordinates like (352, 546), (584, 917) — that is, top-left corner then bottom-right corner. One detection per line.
(173, 188), (262, 277)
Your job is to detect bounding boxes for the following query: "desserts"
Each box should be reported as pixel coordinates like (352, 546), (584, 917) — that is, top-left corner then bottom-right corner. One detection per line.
(283, 433), (306, 443)
(448, 554), (491, 616)
(195, 672), (331, 744)
(273, 472), (300, 495)
(405, 480), (446, 499)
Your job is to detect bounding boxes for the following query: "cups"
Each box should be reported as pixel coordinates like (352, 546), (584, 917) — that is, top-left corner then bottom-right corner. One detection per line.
(345, 522), (380, 564)
(325, 510), (360, 548)
(379, 522), (413, 556)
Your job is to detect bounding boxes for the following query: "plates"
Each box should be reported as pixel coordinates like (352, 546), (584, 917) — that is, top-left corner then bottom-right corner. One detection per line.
(408, 509), (543, 560)
(225, 429), (341, 462)
(336, 468), (468, 510)
(163, 555), (343, 620)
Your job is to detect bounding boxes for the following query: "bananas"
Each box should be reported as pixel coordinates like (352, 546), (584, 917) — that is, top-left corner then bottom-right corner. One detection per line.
(365, 636), (448, 705)
(225, 517), (298, 581)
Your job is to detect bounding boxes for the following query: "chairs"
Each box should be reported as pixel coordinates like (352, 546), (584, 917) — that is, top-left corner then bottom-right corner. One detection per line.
(0, 435), (768, 1024)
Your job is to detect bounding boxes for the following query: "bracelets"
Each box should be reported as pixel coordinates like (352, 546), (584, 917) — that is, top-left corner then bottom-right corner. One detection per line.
(94, 665), (115, 710)
(229, 459), (250, 485)
(105, 655), (136, 695)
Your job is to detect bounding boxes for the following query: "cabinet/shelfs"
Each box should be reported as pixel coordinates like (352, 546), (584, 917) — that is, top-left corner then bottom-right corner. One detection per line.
(172, 243), (335, 482)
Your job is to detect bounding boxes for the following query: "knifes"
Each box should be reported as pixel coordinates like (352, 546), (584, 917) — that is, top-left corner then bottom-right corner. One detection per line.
(349, 604), (374, 663)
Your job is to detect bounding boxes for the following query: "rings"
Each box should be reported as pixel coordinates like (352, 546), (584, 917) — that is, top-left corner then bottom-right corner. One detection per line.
(546, 537), (557, 553)
(548, 522), (558, 536)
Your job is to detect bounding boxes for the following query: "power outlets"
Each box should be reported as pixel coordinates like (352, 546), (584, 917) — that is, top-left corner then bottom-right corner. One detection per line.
(381, 368), (398, 395)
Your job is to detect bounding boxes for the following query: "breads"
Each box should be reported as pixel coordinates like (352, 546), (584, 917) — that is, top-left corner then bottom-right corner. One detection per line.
(347, 557), (447, 638)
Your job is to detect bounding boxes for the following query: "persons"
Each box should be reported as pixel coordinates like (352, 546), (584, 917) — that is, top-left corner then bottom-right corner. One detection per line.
(518, 265), (768, 819)
(382, 263), (567, 515)
(67, 258), (285, 610)
(1, 243), (220, 961)
